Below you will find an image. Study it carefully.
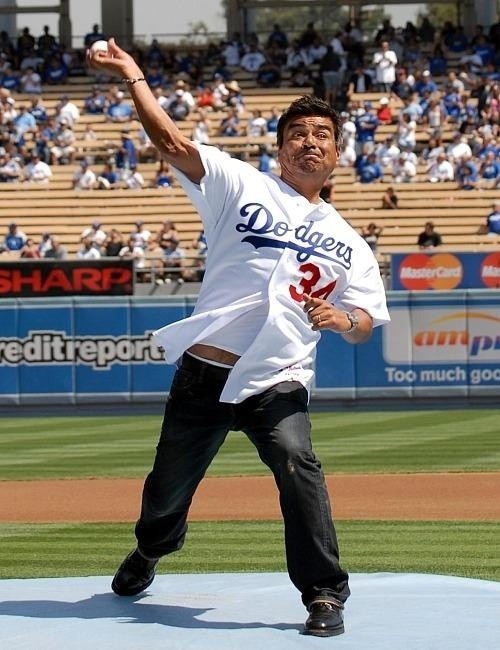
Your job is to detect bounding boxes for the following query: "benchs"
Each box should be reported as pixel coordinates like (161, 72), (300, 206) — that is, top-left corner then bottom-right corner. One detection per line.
(0, 41), (500, 281)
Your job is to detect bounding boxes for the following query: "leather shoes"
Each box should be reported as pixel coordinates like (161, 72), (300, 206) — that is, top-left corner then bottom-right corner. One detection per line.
(110, 545), (159, 597)
(302, 600), (345, 638)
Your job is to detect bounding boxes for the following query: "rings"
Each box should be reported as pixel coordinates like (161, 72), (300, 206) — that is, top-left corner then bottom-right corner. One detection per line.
(318, 314), (321, 322)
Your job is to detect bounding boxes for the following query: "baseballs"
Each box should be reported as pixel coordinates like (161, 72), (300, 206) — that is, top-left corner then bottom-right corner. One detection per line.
(89, 40), (114, 59)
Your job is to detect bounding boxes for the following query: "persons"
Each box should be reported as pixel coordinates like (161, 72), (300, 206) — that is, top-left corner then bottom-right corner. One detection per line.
(83, 33), (392, 638)
(321, 172), (335, 205)
(362, 224), (384, 254)
(382, 187), (398, 209)
(0, 24), (292, 190)
(266, 15), (500, 190)
(4, 221), (207, 284)
(418, 221), (441, 251)
(487, 203), (500, 237)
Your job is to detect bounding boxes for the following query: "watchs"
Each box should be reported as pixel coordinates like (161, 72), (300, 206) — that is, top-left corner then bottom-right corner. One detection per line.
(332, 308), (360, 334)
